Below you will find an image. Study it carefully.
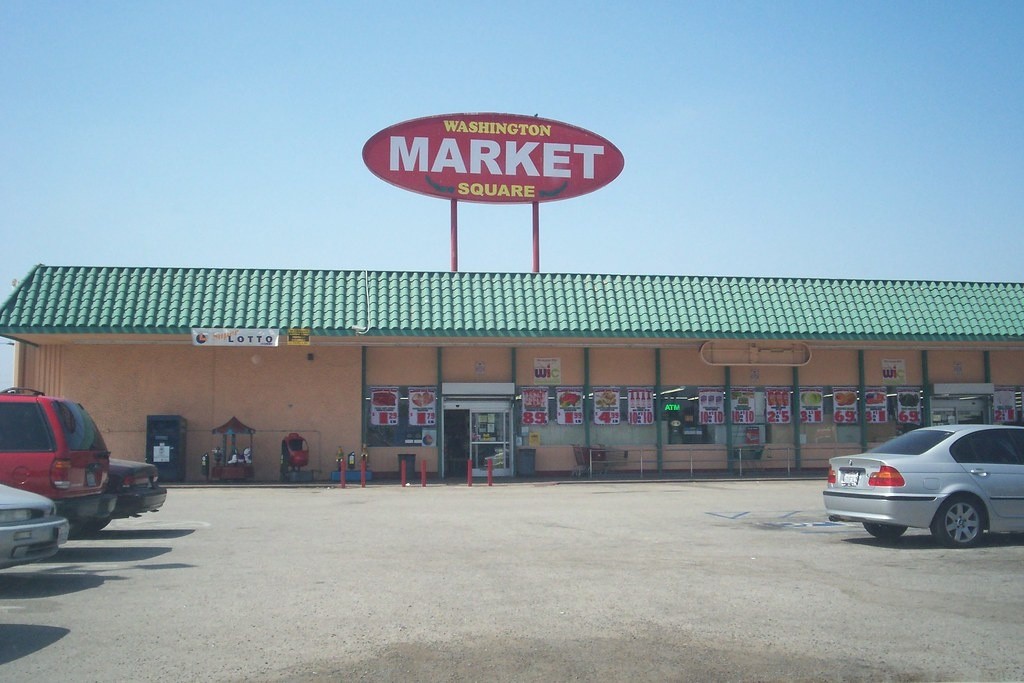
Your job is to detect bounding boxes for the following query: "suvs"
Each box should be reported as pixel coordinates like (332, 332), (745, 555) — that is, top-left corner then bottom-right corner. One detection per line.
(0, 386), (110, 537)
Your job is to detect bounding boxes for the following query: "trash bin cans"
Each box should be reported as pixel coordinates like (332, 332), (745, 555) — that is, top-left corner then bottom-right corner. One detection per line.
(519, 448), (535, 477)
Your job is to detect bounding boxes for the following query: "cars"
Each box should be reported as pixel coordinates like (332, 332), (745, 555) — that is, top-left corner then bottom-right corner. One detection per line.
(0, 484), (70, 570)
(85, 457), (168, 533)
(821, 423), (1024, 550)
